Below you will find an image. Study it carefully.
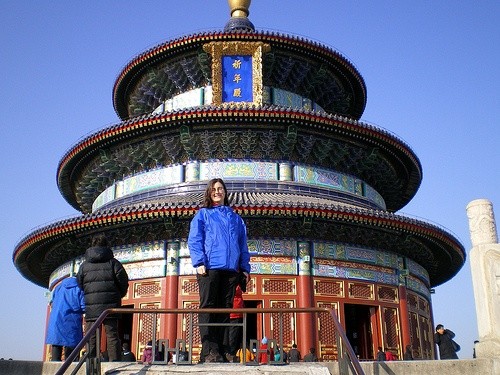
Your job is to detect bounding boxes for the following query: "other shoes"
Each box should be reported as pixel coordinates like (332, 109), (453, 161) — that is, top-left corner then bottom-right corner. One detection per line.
(200, 351), (223, 362)
(223, 352), (239, 363)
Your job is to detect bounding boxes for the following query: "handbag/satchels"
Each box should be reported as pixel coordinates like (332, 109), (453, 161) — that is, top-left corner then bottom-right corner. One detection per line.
(445, 332), (460, 352)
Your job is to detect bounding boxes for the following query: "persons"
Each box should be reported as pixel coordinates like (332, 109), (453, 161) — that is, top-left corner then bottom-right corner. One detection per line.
(188, 177), (251, 364)
(76, 233), (129, 362)
(377, 345), (414, 361)
(120, 343), (136, 362)
(473, 340), (479, 358)
(235, 342), (319, 365)
(141, 339), (189, 364)
(433, 324), (459, 359)
(49, 274), (86, 362)
(223, 284), (244, 363)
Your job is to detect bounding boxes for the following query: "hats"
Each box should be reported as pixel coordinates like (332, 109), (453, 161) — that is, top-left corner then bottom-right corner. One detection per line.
(261, 338), (268, 344)
